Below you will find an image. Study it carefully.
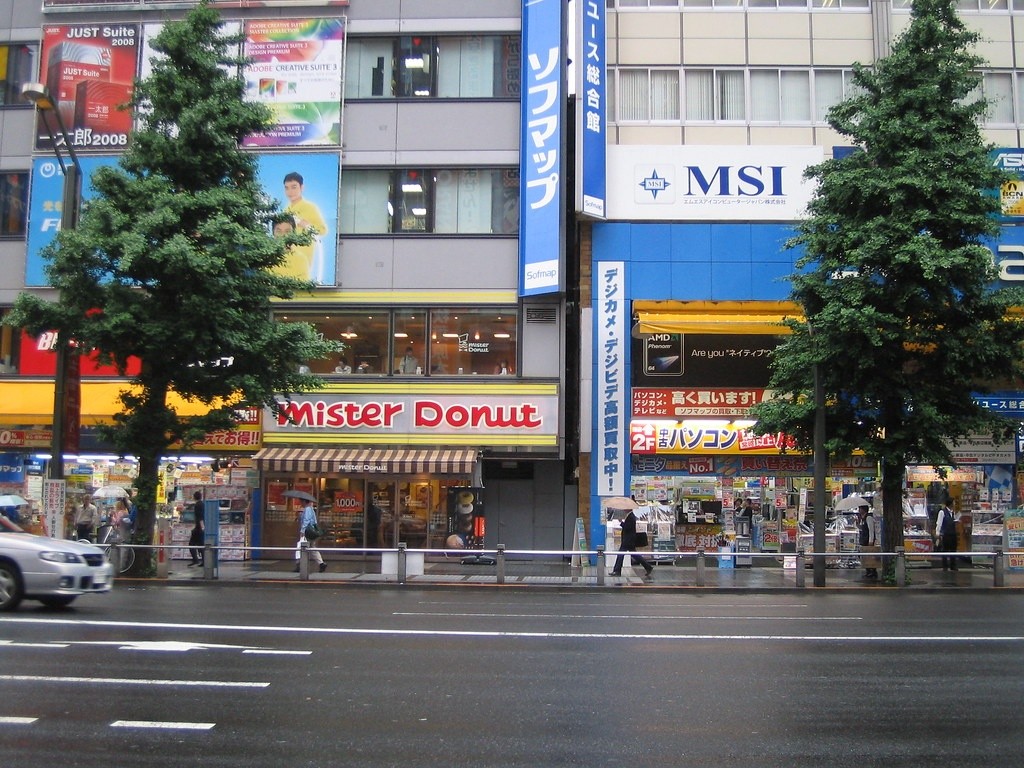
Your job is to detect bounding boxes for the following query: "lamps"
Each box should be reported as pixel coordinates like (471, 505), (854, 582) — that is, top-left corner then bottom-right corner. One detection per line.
(346, 326), (350, 339)
(474, 330), (480, 340)
(340, 327), (358, 338)
(394, 329), (408, 338)
(432, 330), (437, 340)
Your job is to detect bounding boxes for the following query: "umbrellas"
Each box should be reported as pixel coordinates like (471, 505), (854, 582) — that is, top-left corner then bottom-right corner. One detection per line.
(0, 495), (30, 507)
(600, 496), (640, 510)
(833, 496), (872, 511)
(279, 490), (320, 504)
(92, 485), (130, 498)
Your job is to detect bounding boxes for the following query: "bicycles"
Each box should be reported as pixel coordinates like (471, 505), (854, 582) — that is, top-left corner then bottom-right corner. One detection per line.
(77, 524), (136, 574)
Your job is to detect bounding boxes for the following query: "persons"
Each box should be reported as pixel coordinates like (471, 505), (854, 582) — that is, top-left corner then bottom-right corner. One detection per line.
(399, 346), (419, 375)
(734, 497), (753, 536)
(73, 494), (98, 545)
(608, 508), (654, 577)
(0, 492), (20, 532)
(186, 491), (205, 568)
(934, 496), (959, 573)
(687, 501), (699, 513)
(366, 494), (382, 549)
(108, 488), (137, 577)
(355, 358), (376, 375)
(331, 357), (352, 374)
(291, 497), (328, 573)
(493, 358), (510, 376)
(854, 504), (879, 579)
(258, 171), (329, 283)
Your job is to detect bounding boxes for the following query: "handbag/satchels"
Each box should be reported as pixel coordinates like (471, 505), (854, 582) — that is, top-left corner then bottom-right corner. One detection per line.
(304, 521), (324, 540)
(633, 531), (648, 548)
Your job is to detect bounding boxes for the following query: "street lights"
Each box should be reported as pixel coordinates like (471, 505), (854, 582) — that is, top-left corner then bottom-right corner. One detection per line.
(22, 82), (84, 479)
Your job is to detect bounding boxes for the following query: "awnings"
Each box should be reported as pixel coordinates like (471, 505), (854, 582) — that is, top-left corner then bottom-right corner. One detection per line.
(251, 443), (480, 481)
(0, 382), (247, 427)
(630, 298), (1024, 354)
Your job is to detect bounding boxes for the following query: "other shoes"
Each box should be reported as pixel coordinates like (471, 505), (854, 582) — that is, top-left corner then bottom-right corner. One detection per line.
(950, 568), (958, 571)
(942, 568), (947, 572)
(188, 561), (198, 567)
(608, 571), (621, 576)
(292, 567), (300, 572)
(318, 562), (328, 573)
(864, 571), (877, 577)
(198, 563), (204, 568)
(645, 566), (654, 576)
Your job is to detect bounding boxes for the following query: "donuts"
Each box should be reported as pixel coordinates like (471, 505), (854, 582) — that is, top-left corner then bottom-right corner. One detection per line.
(455, 491), (473, 533)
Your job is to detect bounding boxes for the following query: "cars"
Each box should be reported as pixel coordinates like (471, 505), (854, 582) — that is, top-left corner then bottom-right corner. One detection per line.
(0, 512), (114, 612)
